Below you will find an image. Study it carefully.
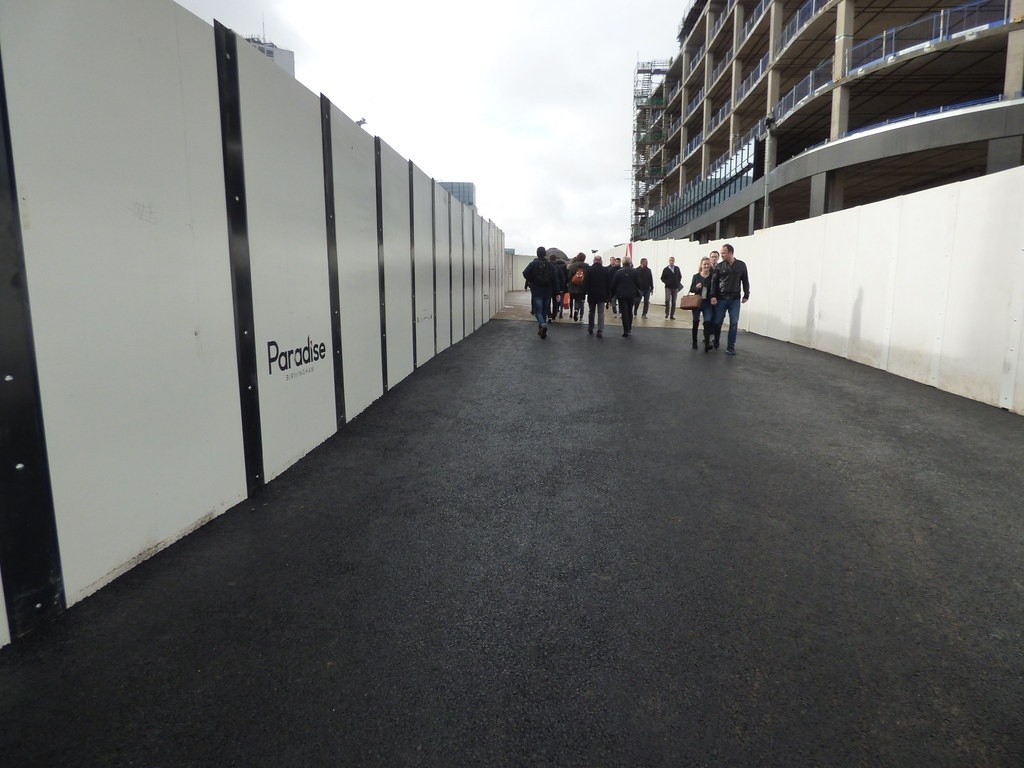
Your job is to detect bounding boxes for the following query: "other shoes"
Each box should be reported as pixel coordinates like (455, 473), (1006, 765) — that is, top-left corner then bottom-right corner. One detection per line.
(597, 331), (602, 338)
(559, 312), (562, 318)
(622, 332), (628, 337)
(714, 337), (720, 349)
(613, 314), (617, 318)
(628, 326), (631, 332)
(538, 326), (547, 339)
(548, 318), (552, 323)
(606, 303), (609, 309)
(726, 348), (736, 355)
(579, 318), (584, 321)
(588, 329), (593, 334)
(642, 314), (647, 319)
(665, 314), (668, 318)
(670, 316), (675, 320)
(620, 314), (622, 317)
(574, 310), (578, 321)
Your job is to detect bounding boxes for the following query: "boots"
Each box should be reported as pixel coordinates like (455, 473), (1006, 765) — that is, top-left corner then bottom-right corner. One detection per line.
(692, 320), (700, 349)
(704, 321), (710, 352)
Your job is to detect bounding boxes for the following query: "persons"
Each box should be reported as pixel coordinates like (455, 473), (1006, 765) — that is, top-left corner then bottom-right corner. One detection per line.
(568, 253), (588, 321)
(607, 257), (615, 268)
(583, 255), (608, 338)
(565, 257), (576, 317)
(610, 256), (641, 337)
(688, 257), (713, 352)
(710, 244), (750, 354)
(548, 254), (567, 323)
(634, 258), (653, 318)
(660, 257), (682, 320)
(710, 251), (719, 267)
(522, 246), (555, 340)
(608, 258), (622, 318)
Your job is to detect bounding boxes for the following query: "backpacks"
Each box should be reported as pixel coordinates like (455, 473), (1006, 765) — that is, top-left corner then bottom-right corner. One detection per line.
(571, 267), (584, 285)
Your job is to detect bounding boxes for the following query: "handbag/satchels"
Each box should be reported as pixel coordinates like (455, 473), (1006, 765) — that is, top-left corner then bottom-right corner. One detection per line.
(563, 292), (571, 309)
(680, 291), (702, 310)
